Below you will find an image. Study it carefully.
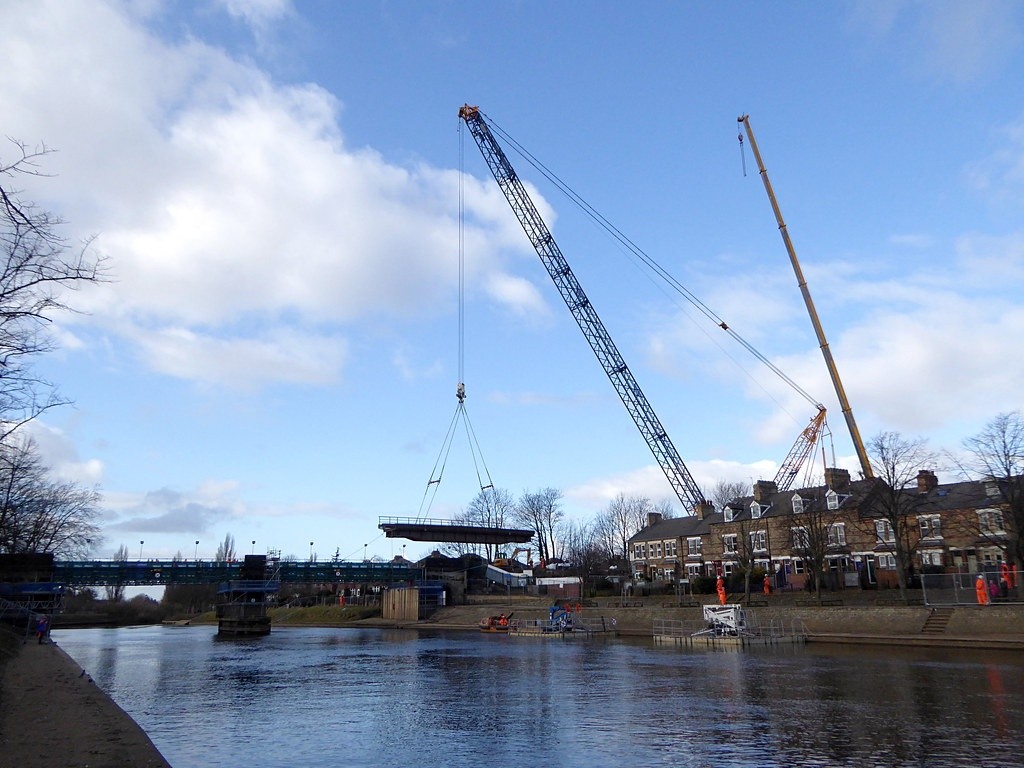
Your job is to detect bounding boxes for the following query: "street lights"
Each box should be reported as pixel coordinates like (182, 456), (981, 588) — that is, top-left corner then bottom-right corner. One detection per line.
(310, 542), (314, 561)
(364, 544), (367, 560)
(251, 540), (255, 555)
(138, 540), (145, 562)
(402, 545), (407, 559)
(195, 540), (201, 560)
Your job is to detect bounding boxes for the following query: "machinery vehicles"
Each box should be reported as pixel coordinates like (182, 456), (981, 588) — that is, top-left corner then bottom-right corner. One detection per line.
(494, 546), (534, 569)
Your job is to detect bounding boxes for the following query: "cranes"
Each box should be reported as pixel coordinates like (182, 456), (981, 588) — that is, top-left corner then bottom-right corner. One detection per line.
(457, 99), (827, 518)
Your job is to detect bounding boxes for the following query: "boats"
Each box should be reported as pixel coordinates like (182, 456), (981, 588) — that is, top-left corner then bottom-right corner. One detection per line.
(478, 610), (517, 632)
(544, 606), (593, 632)
(377, 515), (535, 545)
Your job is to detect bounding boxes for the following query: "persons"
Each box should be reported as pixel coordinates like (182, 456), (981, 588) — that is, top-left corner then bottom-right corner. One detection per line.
(340, 595), (345, 605)
(1001, 559), (1020, 590)
(611, 617), (617, 630)
(988, 580), (998, 597)
(1000, 578), (1008, 597)
(763, 574), (769, 595)
(37, 614), (49, 644)
(575, 603), (581, 618)
(226, 557), (238, 563)
(530, 559), (533, 568)
(716, 575), (726, 605)
(976, 574), (987, 605)
(541, 556), (545, 568)
(565, 603), (571, 618)
(498, 613), (507, 626)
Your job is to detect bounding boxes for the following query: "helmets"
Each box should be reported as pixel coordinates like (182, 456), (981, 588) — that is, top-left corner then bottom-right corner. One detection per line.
(979, 575), (983, 579)
(765, 574), (768, 576)
(1002, 561), (1006, 563)
(717, 576), (720, 580)
